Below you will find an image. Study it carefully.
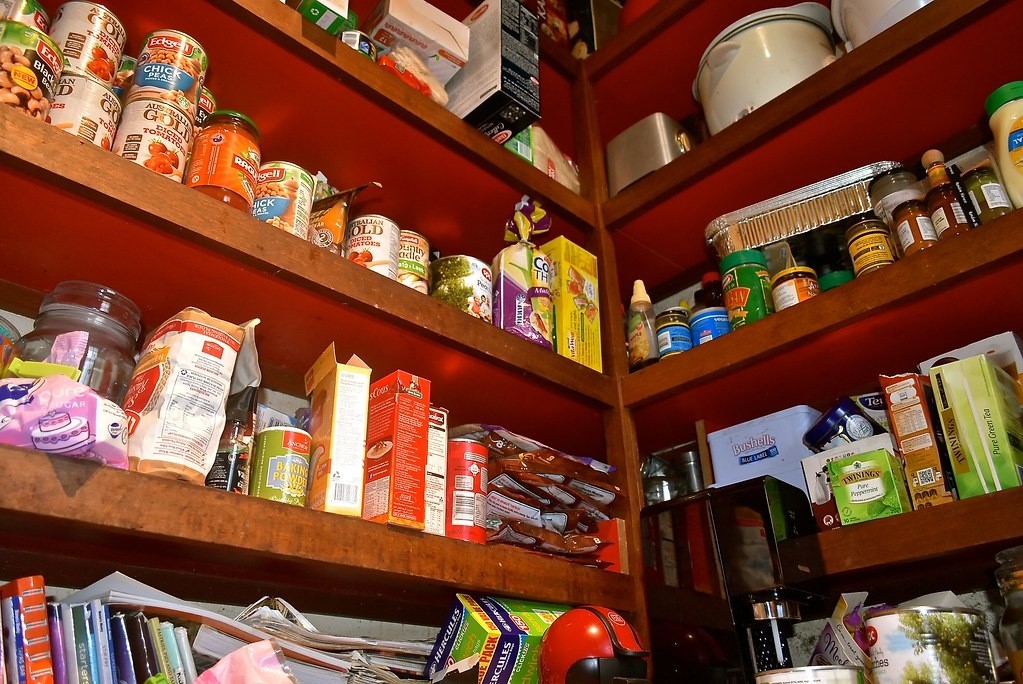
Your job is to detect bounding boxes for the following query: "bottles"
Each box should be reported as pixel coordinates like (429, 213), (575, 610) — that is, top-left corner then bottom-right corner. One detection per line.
(868, 167), (927, 260)
(680, 205), (902, 367)
(654, 310), (692, 360)
(984, 80), (1023, 209)
(928, 184), (970, 241)
(994, 545), (1023, 684)
(892, 198), (937, 256)
(627, 280), (658, 373)
(961, 167), (1014, 224)
(0, 281), (141, 407)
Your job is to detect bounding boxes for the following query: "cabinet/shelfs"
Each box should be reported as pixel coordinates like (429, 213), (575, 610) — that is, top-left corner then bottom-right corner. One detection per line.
(0, 0), (1023, 684)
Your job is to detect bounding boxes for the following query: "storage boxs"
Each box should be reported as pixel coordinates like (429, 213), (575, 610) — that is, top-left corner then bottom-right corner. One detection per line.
(504, 124), (535, 166)
(929, 354), (1023, 499)
(422, 403), (448, 536)
(358, 0), (471, 86)
(799, 433), (915, 531)
(304, 341), (372, 516)
(878, 373), (960, 511)
(539, 235), (602, 373)
(705, 405), (824, 486)
(849, 392), (895, 436)
(422, 593), (574, 684)
(826, 448), (913, 526)
(444, 0), (542, 147)
(806, 591), (875, 684)
(296, 0), (350, 36)
(360, 370), (432, 532)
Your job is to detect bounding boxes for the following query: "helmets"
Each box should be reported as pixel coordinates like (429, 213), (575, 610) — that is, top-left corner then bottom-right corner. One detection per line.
(537, 606), (650, 684)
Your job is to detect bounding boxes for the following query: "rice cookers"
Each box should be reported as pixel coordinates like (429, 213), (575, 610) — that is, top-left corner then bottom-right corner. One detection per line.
(692, 0), (934, 135)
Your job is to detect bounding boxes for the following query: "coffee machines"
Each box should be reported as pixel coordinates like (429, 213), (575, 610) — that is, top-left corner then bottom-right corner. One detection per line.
(639, 475), (829, 684)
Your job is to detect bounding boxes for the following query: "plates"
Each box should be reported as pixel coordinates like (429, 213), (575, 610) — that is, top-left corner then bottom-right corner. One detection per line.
(367, 441), (393, 458)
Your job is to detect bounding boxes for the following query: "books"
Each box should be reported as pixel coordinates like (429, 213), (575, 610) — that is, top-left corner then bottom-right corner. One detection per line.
(1, 575), (199, 684)
(61, 570), (434, 683)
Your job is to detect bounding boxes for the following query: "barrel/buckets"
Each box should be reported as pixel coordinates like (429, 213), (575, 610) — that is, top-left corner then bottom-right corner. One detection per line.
(754, 666), (859, 684)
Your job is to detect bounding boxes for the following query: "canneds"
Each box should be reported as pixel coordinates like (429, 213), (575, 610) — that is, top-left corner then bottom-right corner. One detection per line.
(861, 603), (998, 684)
(770, 266), (821, 313)
(252, 426), (313, 508)
(655, 310), (693, 361)
(0, 0), (495, 325)
(843, 166), (1014, 280)
